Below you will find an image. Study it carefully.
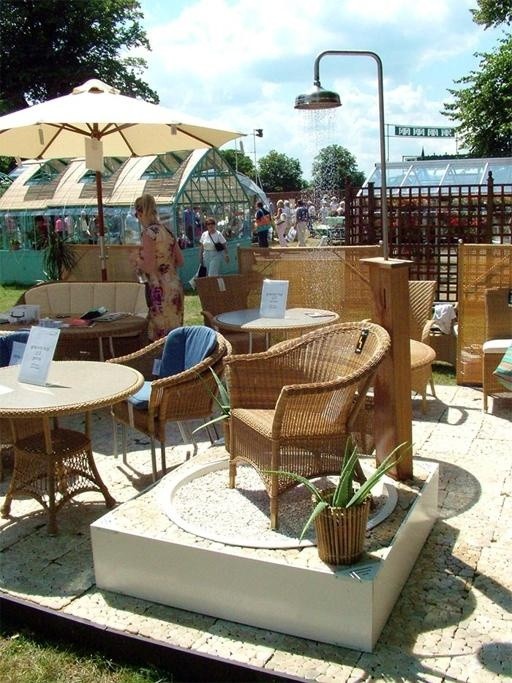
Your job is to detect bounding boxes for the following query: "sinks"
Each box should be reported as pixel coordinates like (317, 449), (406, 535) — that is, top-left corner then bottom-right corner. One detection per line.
(13, 280), (151, 362)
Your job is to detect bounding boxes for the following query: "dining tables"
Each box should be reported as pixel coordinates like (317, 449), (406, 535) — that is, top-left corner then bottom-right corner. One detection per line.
(264, 437), (416, 565)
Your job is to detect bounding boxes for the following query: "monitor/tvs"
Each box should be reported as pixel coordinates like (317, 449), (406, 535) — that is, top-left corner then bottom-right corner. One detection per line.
(69, 318), (97, 329)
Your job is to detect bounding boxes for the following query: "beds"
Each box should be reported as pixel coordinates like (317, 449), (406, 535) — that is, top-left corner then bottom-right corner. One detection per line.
(0, 76), (248, 283)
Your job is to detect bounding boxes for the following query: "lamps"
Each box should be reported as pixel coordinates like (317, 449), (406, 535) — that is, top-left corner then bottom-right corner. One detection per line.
(222, 321), (391, 531)
(483, 352), (512, 414)
(409, 280), (439, 346)
(103, 324), (232, 484)
(429, 304), (457, 364)
(192, 273), (266, 355)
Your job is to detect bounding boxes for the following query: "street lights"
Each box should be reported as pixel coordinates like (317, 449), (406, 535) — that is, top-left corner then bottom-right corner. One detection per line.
(215, 243), (224, 252)
(255, 214), (271, 232)
(198, 264), (208, 278)
(275, 218), (283, 224)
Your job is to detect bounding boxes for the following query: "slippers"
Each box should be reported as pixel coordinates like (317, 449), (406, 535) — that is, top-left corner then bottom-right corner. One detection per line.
(298, 206), (308, 220)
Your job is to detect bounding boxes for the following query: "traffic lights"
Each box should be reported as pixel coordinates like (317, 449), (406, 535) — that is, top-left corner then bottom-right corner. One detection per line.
(491, 344), (512, 391)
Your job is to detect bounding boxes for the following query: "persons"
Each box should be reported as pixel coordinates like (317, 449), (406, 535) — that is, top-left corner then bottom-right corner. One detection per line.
(184, 205), (203, 246)
(127, 193), (184, 345)
(254, 194), (345, 247)
(5, 208), (99, 250)
(199, 217), (229, 277)
